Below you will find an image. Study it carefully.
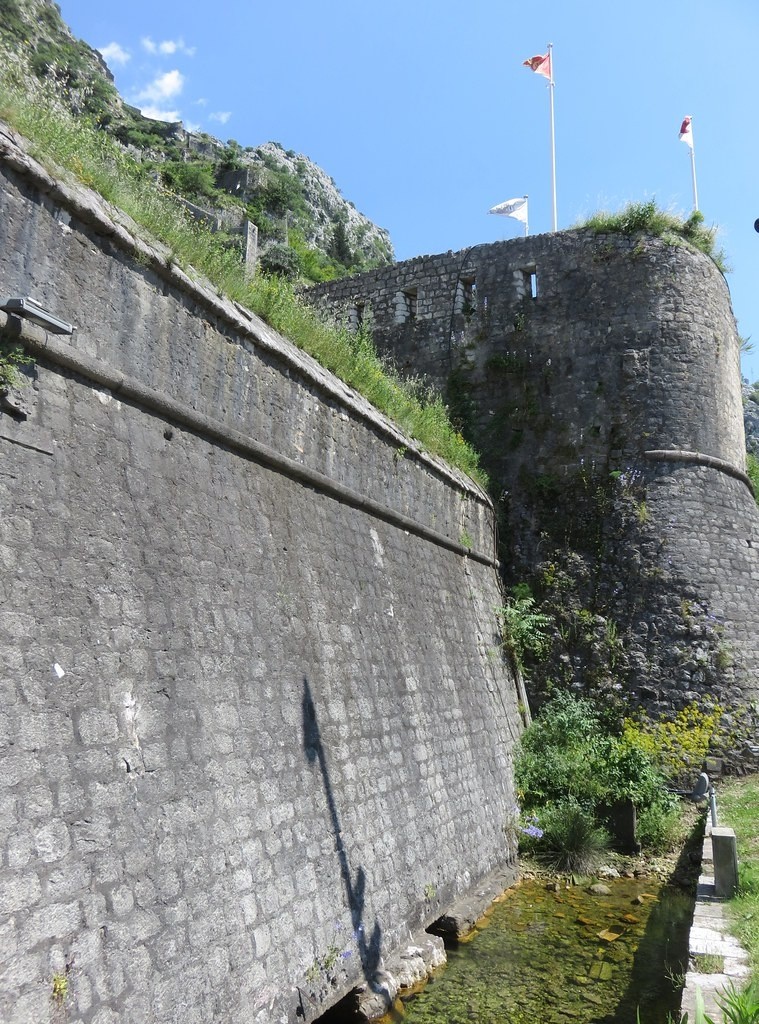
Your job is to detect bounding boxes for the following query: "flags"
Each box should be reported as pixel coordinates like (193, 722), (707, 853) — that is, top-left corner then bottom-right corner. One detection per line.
(487, 197), (528, 224)
(522, 52), (551, 80)
(677, 116), (693, 149)
